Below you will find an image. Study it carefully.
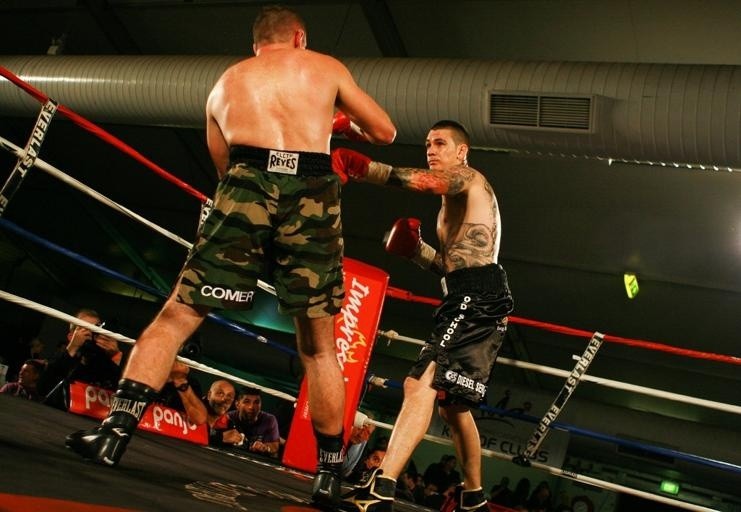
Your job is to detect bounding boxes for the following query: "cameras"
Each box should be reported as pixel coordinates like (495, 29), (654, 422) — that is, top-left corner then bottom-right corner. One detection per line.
(181, 342), (200, 359)
(90, 320), (106, 336)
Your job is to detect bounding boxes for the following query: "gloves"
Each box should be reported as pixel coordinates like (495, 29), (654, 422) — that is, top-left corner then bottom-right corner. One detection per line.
(383, 216), (436, 272)
(331, 108), (382, 146)
(330, 147), (394, 187)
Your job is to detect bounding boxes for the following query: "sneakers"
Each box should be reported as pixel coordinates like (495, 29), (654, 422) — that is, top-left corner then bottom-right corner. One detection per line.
(340, 466), (400, 512)
(310, 463), (344, 506)
(62, 412), (139, 468)
(449, 483), (491, 511)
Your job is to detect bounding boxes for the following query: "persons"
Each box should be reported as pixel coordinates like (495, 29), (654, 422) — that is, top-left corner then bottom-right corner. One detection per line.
(337, 407), (573, 512)
(65, 5), (397, 502)
(331, 121), (515, 512)
(1, 308), (280, 458)
